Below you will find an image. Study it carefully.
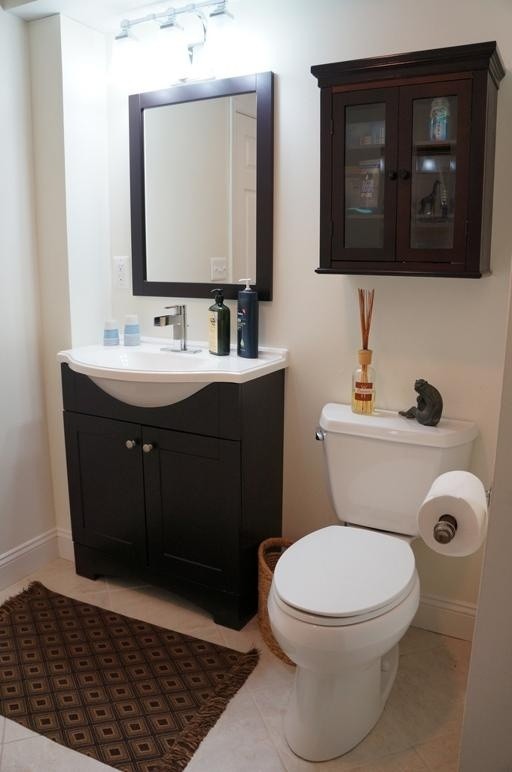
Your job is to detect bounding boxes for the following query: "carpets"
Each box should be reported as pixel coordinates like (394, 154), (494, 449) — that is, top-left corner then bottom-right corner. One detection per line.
(0, 577), (260, 771)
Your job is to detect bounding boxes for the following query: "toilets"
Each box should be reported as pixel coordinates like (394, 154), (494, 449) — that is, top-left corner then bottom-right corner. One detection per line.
(267, 403), (478, 762)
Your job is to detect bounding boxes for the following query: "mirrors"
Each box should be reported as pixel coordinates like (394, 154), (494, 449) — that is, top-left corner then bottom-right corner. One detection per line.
(128, 71), (274, 302)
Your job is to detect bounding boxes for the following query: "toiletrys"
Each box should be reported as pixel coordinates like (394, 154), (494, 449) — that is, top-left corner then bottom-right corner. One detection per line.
(103, 320), (119, 345)
(124, 314), (139, 346)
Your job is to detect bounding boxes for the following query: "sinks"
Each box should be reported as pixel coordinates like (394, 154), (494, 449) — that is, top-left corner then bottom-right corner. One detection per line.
(76, 349), (206, 372)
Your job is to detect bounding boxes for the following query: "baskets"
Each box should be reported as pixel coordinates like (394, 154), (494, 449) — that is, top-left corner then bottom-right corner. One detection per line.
(257, 537), (298, 666)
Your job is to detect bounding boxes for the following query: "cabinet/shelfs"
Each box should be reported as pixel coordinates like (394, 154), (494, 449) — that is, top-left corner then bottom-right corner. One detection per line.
(60, 362), (286, 631)
(311, 39), (505, 279)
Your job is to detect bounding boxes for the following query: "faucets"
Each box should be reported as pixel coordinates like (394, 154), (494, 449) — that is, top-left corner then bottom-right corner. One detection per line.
(153, 305), (187, 350)
